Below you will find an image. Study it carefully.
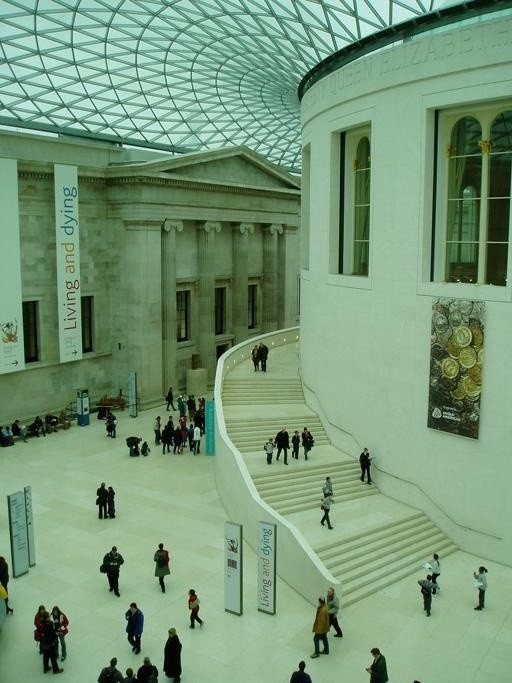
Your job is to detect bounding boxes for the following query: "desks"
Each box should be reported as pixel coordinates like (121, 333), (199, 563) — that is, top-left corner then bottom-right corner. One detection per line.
(91, 398), (129, 420)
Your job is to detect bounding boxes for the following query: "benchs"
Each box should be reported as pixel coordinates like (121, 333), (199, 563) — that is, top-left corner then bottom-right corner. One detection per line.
(12, 415), (73, 442)
